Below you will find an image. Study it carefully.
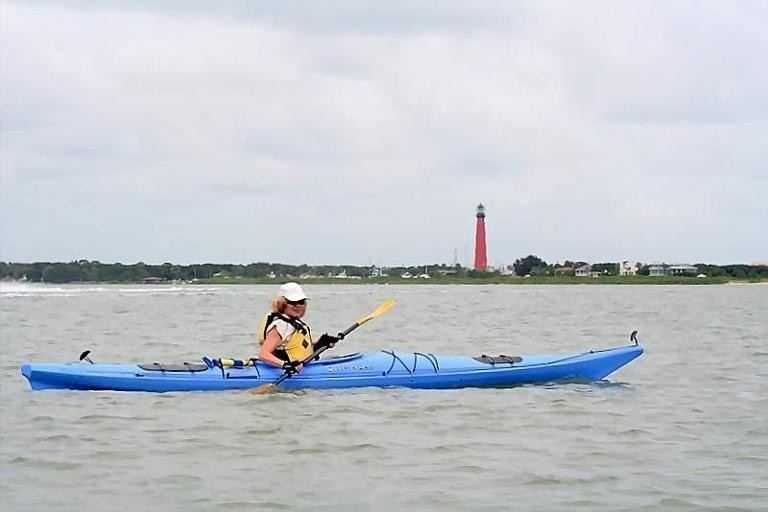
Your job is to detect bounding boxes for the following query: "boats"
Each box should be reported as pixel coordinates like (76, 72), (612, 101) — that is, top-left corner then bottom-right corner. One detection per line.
(21, 329), (645, 391)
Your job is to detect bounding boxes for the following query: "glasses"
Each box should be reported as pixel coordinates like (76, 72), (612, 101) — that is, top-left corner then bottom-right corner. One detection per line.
(287, 300), (306, 305)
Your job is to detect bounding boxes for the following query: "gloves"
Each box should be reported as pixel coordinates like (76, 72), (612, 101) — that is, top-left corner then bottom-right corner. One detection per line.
(321, 335), (339, 347)
(283, 361), (303, 372)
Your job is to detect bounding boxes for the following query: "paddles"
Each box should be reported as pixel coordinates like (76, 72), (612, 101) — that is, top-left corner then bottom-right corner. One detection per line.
(254, 299), (396, 395)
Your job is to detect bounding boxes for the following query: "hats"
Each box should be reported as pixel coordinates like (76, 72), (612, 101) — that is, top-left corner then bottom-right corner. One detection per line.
(280, 282), (309, 301)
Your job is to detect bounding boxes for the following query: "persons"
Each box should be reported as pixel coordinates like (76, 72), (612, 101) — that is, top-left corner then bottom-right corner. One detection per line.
(256, 281), (340, 374)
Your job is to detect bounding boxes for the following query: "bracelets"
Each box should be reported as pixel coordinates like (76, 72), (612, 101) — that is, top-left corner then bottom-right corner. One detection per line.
(281, 361), (294, 371)
